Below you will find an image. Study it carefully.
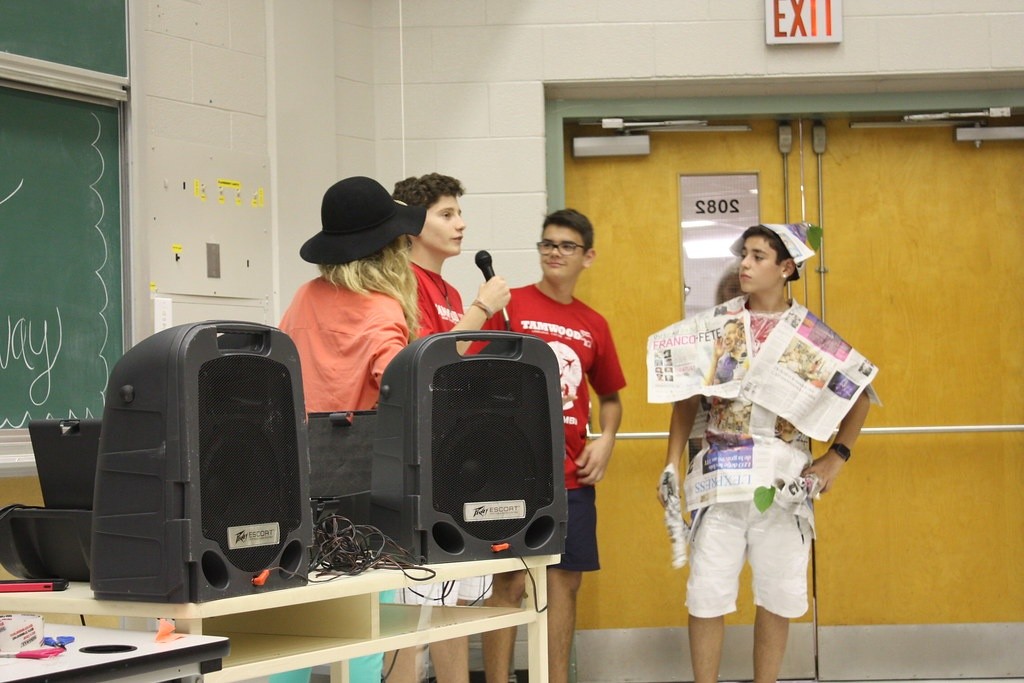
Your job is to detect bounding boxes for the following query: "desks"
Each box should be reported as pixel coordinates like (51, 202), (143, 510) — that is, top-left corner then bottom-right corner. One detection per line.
(0, 552), (565, 682)
(0, 609), (230, 683)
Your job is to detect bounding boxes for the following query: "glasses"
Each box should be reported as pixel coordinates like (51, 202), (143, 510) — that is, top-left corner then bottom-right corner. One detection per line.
(537, 241), (588, 256)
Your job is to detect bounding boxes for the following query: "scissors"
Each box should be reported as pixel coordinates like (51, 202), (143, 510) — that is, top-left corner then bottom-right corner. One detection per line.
(44, 636), (75, 652)
(0, 648), (65, 660)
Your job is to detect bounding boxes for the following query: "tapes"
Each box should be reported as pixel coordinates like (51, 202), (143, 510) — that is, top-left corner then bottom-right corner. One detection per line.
(0, 612), (44, 656)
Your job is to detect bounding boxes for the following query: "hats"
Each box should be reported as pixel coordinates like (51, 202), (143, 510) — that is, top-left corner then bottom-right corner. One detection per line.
(299, 176), (427, 266)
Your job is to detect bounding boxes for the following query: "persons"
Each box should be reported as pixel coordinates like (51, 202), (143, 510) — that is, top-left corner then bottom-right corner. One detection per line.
(277, 174), (415, 683)
(468, 207), (628, 683)
(391, 173), (512, 683)
(658, 224), (871, 683)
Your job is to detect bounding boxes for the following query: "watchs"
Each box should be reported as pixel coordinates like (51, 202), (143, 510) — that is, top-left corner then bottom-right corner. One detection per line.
(827, 442), (852, 464)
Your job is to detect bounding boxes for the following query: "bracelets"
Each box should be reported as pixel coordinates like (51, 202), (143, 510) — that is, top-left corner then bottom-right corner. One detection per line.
(471, 299), (495, 320)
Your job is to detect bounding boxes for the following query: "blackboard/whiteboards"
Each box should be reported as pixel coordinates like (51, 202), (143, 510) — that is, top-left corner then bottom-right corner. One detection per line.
(0, 0), (133, 478)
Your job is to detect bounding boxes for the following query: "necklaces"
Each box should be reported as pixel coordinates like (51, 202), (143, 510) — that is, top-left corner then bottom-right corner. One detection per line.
(410, 261), (452, 310)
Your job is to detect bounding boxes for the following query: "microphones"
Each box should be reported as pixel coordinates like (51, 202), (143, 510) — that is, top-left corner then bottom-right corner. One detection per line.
(476, 250), (511, 327)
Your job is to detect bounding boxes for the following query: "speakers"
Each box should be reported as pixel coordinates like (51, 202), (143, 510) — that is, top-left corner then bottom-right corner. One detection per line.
(368, 330), (570, 563)
(89, 319), (314, 606)
